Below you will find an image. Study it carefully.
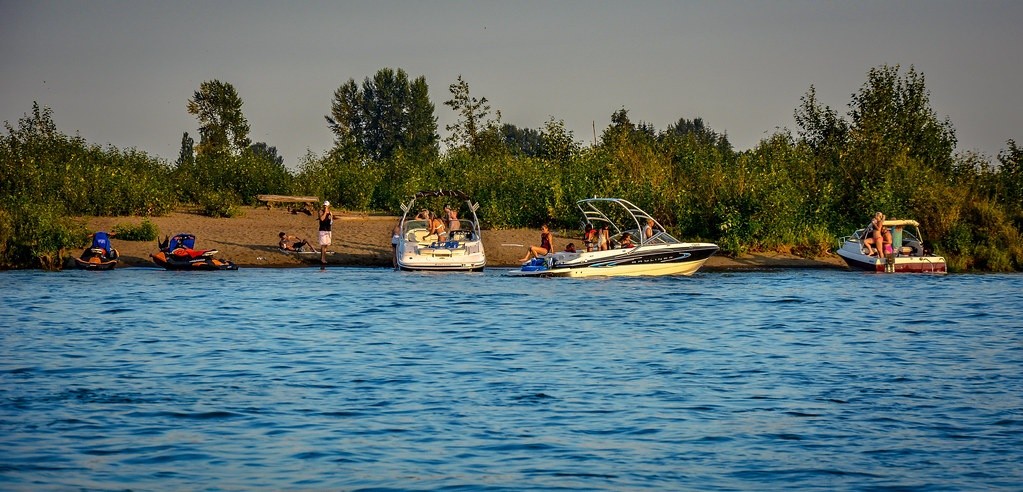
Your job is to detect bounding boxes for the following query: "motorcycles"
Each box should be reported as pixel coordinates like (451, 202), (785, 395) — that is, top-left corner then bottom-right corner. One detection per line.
(149, 233), (238, 270)
(72, 232), (121, 271)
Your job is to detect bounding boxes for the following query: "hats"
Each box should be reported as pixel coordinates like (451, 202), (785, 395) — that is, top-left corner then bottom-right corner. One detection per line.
(323, 201), (330, 206)
(443, 204), (451, 210)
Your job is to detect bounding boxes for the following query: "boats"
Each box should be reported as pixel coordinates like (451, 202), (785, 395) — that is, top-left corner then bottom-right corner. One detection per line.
(508, 195), (719, 277)
(836, 219), (950, 278)
(391, 189), (487, 272)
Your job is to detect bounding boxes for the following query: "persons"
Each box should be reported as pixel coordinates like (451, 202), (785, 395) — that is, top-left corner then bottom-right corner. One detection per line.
(415, 204), (460, 242)
(422, 211), (448, 249)
(880, 226), (893, 258)
(254, 199), (314, 224)
(279, 232), (318, 253)
(563, 243), (577, 253)
(579, 223), (634, 252)
(864, 212), (885, 258)
(518, 224), (554, 262)
(641, 217), (655, 245)
(318, 200), (333, 263)
(392, 226), (401, 271)
